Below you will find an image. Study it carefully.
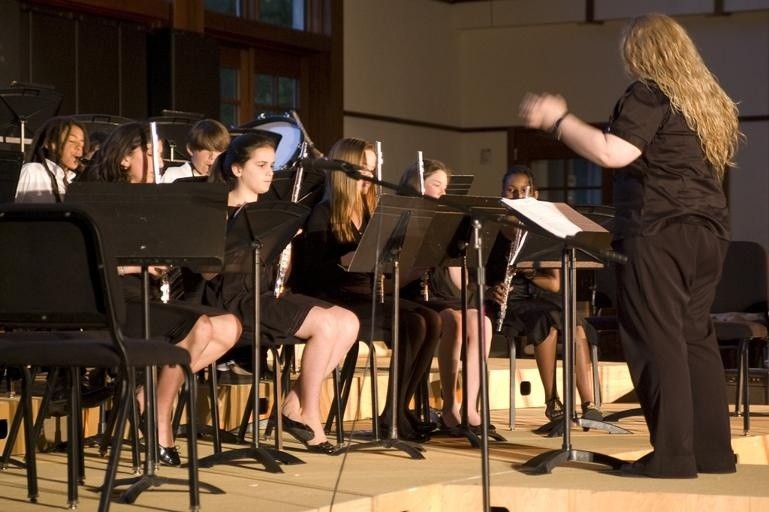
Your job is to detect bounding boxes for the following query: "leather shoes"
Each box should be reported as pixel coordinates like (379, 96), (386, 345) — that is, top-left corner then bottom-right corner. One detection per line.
(301, 441), (335, 454)
(268, 409), (314, 441)
(459, 408), (496, 433)
(441, 416), (464, 438)
(378, 415), (437, 444)
(139, 411), (180, 467)
(581, 402), (603, 431)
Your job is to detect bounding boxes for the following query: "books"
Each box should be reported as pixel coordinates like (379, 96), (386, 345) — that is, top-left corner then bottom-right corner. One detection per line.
(501, 197), (610, 240)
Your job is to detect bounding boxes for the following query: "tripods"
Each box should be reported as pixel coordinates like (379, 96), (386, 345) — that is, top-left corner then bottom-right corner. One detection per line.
(101, 280), (632, 504)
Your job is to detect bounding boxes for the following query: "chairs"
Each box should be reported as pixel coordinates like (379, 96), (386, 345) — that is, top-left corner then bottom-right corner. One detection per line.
(708, 241), (768, 435)
(0, 202), (633, 511)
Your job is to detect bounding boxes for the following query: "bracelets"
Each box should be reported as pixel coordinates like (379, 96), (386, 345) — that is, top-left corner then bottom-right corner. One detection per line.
(549, 110), (571, 136)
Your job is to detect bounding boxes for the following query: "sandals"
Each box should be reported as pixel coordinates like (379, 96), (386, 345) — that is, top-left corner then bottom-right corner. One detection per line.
(545, 398), (564, 421)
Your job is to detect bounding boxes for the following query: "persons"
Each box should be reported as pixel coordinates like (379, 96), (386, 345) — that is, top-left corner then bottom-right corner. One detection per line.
(159, 118), (360, 452)
(396, 158), (495, 437)
(518, 15), (740, 479)
(298, 138), (442, 443)
(486, 167), (603, 422)
(13, 118), (244, 464)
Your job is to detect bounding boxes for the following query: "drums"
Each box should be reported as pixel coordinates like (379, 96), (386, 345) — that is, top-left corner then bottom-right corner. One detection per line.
(231, 112), (315, 171)
